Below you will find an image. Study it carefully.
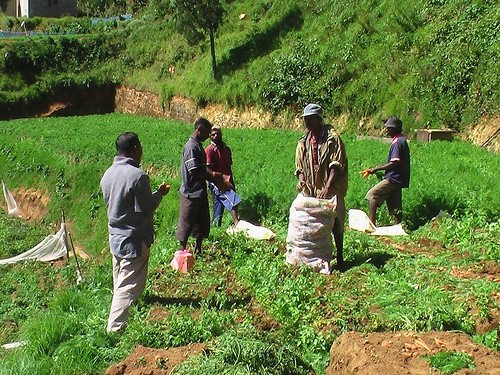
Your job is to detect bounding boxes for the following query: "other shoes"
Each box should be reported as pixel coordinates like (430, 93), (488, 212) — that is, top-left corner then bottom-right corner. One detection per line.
(337, 261), (347, 271)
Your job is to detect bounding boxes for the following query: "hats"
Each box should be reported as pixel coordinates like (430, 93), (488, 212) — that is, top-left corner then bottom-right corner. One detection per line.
(211, 124), (221, 130)
(384, 116), (402, 130)
(300, 103), (324, 119)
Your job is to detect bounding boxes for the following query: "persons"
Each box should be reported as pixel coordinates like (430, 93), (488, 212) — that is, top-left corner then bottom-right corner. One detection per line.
(294, 104), (348, 270)
(360, 118), (410, 231)
(99, 132), (170, 332)
(176, 118), (241, 255)
